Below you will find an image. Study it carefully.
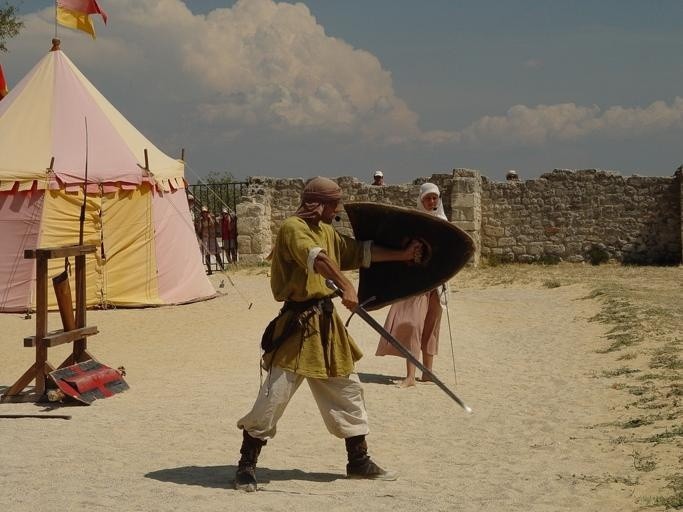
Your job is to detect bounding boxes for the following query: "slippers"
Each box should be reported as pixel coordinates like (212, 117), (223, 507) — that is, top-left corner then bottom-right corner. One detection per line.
(397, 380), (416, 390)
(421, 375), (432, 383)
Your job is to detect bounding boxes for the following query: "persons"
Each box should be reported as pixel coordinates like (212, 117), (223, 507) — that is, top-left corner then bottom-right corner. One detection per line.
(371, 170), (385, 186)
(188, 194), (237, 275)
(398, 182), (448, 389)
(503, 170), (519, 181)
(236, 177), (423, 493)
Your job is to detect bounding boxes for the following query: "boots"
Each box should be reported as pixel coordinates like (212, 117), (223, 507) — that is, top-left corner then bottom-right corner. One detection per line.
(234, 428), (267, 492)
(344, 435), (400, 480)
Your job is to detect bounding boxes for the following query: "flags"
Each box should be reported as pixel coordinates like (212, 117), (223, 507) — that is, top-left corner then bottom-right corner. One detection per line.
(56, 0), (109, 41)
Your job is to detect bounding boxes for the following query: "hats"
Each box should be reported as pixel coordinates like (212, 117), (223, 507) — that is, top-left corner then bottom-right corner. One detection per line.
(374, 171), (383, 177)
(202, 207), (207, 212)
(301, 177), (343, 201)
(188, 195), (194, 200)
(505, 170), (518, 178)
(222, 209), (227, 214)
(229, 213), (236, 220)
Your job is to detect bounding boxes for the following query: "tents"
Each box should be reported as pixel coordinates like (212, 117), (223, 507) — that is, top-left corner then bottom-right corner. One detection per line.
(0, 49), (218, 308)
